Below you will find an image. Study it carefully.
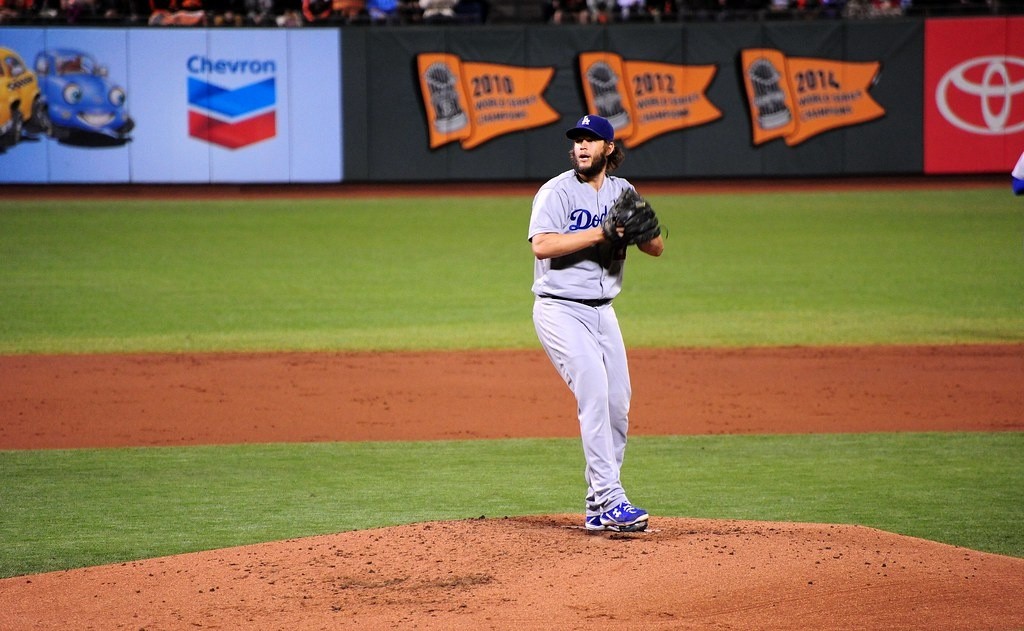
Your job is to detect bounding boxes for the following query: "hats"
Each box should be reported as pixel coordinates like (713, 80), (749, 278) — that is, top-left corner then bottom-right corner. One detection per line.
(566, 115), (614, 140)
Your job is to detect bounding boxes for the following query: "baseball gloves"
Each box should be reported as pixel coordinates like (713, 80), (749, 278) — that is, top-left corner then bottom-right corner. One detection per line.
(600, 187), (669, 247)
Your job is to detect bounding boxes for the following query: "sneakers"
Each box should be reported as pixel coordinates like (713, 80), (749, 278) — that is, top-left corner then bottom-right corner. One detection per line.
(600, 501), (648, 526)
(585, 515), (648, 531)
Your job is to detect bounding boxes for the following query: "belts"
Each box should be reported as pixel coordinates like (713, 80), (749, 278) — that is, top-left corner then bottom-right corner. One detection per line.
(539, 295), (611, 307)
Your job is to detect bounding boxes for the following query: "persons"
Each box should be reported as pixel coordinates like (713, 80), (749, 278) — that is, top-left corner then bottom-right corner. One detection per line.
(528, 115), (664, 532)
(0, 0), (827, 26)
